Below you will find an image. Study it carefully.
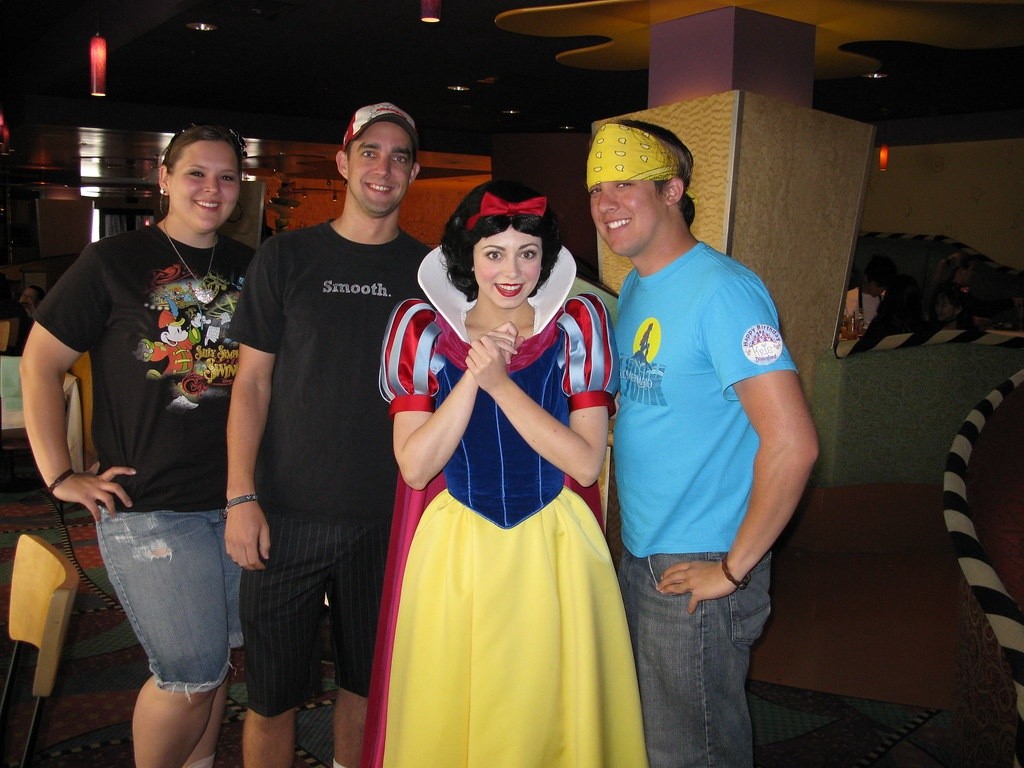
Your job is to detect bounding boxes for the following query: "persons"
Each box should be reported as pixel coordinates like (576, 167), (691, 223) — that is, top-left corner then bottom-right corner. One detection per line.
(587, 123), (819, 768)
(868, 274), (934, 331)
(843, 255), (898, 324)
(221, 104), (432, 768)
(927, 250), (1024, 330)
(0, 272), (45, 356)
(359, 177), (645, 768)
(19, 126), (255, 768)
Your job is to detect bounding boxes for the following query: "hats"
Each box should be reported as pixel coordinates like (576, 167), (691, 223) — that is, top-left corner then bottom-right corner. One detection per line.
(342, 102), (419, 152)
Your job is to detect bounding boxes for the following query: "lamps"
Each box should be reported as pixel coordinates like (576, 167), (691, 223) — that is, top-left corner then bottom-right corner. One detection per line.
(87, 0), (109, 97)
(878, 105), (892, 171)
(418, 0), (442, 23)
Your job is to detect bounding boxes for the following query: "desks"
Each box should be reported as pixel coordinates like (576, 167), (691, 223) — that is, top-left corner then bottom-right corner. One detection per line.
(835, 333), (862, 358)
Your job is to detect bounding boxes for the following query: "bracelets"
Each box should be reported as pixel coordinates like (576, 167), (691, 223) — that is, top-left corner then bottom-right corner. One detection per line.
(47, 469), (74, 493)
(223, 494), (258, 517)
(721, 556), (751, 589)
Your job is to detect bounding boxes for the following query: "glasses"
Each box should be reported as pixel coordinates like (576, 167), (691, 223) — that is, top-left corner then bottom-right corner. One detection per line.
(162, 120), (247, 165)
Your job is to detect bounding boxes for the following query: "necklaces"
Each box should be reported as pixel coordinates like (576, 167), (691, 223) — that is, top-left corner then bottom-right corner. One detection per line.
(163, 216), (217, 304)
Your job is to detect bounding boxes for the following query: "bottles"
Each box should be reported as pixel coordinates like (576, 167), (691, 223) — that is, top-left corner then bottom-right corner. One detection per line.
(841, 311), (865, 341)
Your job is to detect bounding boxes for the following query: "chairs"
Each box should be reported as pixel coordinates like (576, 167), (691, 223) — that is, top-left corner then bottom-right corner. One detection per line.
(0, 351), (84, 527)
(0, 534), (79, 768)
